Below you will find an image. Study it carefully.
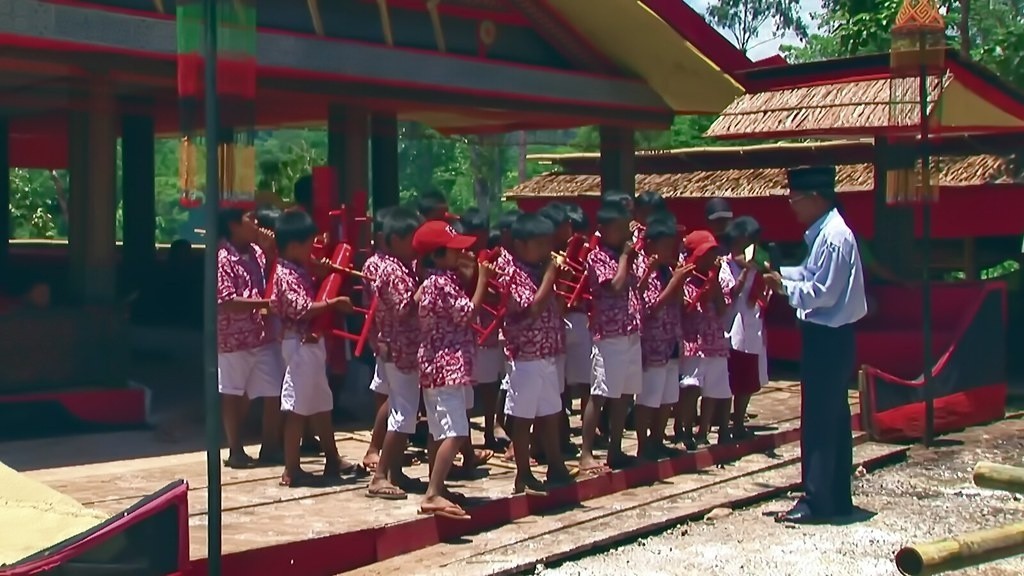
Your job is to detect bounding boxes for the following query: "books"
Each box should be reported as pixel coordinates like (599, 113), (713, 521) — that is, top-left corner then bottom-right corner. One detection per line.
(744, 243), (779, 275)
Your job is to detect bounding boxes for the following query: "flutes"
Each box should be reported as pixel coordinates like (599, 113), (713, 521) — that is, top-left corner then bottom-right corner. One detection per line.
(310, 258), (375, 281)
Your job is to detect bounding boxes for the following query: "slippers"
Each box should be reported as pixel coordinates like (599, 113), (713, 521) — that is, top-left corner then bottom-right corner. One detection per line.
(279, 462), (370, 486)
(430, 449), (494, 478)
(363, 450), (421, 472)
(365, 475), (421, 499)
(579, 461), (612, 475)
(417, 492), (471, 519)
(500, 455), (539, 467)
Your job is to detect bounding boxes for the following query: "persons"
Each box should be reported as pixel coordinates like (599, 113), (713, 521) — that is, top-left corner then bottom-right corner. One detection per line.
(151, 237), (204, 330)
(211, 190), (769, 499)
(493, 212), (599, 497)
(413, 220), (496, 520)
(736, 164), (868, 522)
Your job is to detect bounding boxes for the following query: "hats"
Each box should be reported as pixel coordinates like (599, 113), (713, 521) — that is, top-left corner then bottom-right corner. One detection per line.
(412, 220), (477, 256)
(787, 165), (836, 190)
(684, 231), (719, 256)
(706, 198), (732, 220)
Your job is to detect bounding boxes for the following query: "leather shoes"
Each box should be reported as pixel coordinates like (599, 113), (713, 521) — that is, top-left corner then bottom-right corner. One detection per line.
(774, 500), (853, 524)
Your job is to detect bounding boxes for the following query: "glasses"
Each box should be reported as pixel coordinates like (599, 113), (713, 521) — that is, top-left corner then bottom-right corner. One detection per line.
(789, 195), (809, 204)
